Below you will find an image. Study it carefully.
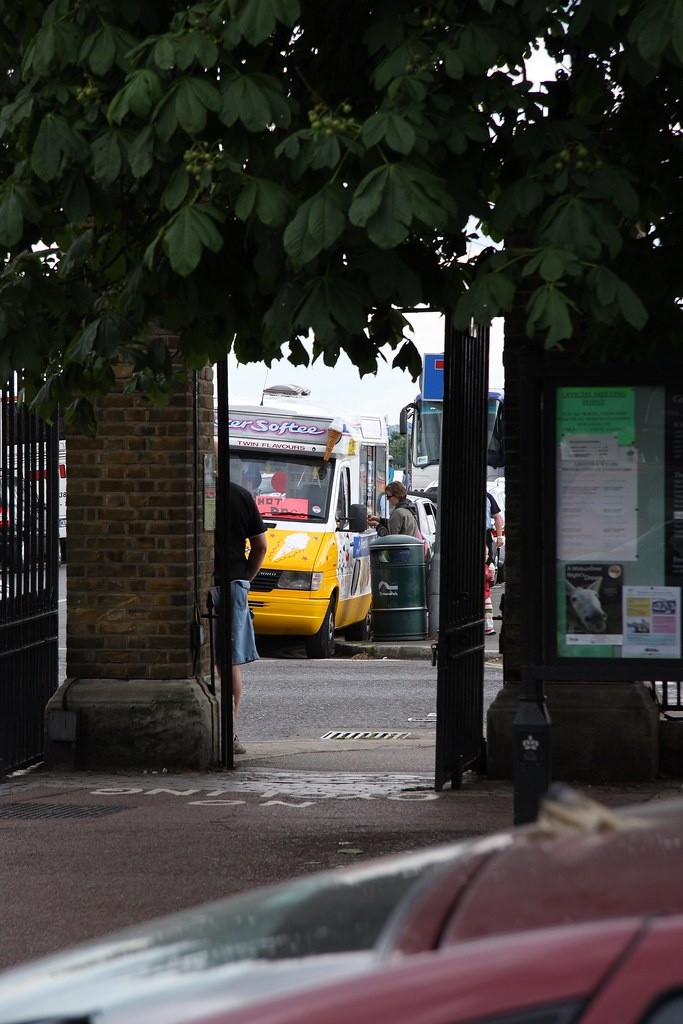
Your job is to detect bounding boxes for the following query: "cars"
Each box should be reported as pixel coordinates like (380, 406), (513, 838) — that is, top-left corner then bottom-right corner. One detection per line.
(406, 493), (438, 550)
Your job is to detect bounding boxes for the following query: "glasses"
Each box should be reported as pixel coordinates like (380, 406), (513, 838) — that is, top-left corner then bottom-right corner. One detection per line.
(387, 495), (394, 500)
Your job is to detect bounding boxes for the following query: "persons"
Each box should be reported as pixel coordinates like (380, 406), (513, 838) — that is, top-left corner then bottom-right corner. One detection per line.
(215, 475), (267, 755)
(389, 455), (394, 483)
(367, 481), (418, 562)
(485, 492), (503, 635)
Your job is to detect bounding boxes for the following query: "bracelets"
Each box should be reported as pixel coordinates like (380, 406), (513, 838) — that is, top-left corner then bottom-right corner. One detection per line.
(497, 536), (502, 539)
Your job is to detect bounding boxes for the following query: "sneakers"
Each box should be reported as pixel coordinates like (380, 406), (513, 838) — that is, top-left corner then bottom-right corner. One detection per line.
(483, 628), (497, 635)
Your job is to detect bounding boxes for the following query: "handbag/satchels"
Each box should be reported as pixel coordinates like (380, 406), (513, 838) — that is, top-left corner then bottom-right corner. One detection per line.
(417, 529), (428, 561)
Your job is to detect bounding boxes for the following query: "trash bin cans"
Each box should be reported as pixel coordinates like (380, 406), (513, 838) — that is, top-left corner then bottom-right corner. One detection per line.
(367, 536), (429, 644)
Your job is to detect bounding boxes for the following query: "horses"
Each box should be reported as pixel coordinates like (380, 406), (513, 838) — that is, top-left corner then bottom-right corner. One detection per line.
(566, 577), (608, 635)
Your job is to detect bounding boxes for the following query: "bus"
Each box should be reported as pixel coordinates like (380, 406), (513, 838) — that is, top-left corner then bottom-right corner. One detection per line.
(211, 384), (395, 659)
(398, 386), (508, 583)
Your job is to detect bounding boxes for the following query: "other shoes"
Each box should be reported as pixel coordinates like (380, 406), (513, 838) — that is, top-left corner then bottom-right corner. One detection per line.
(233, 734), (247, 754)
